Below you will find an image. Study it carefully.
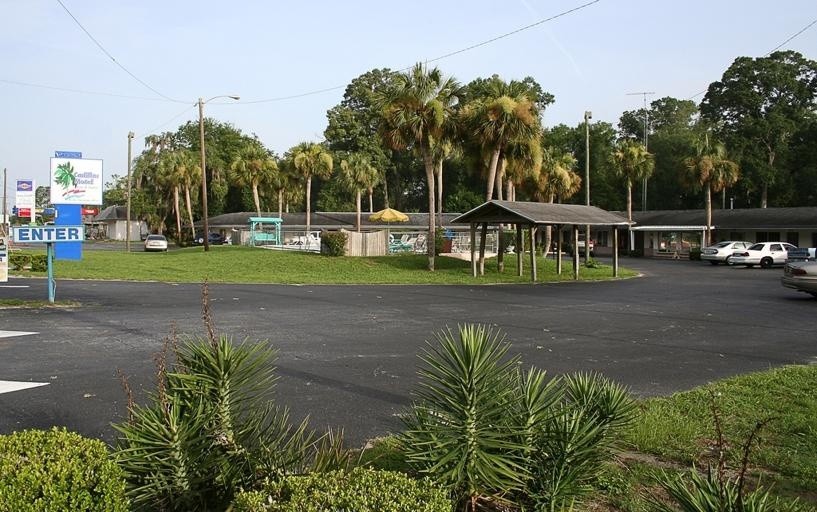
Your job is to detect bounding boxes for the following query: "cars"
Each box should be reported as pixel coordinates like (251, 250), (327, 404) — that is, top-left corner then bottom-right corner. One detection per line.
(192, 233), (224, 246)
(780, 260), (817, 297)
(729, 242), (797, 269)
(699, 240), (753, 266)
(144, 234), (167, 252)
(292, 231), (322, 245)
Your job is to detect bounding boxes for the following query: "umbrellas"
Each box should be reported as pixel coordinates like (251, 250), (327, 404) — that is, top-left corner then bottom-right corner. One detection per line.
(368, 207), (409, 243)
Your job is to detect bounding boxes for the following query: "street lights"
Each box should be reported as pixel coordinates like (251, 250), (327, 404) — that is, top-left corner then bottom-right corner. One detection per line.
(195, 95), (242, 251)
(584, 111), (593, 266)
